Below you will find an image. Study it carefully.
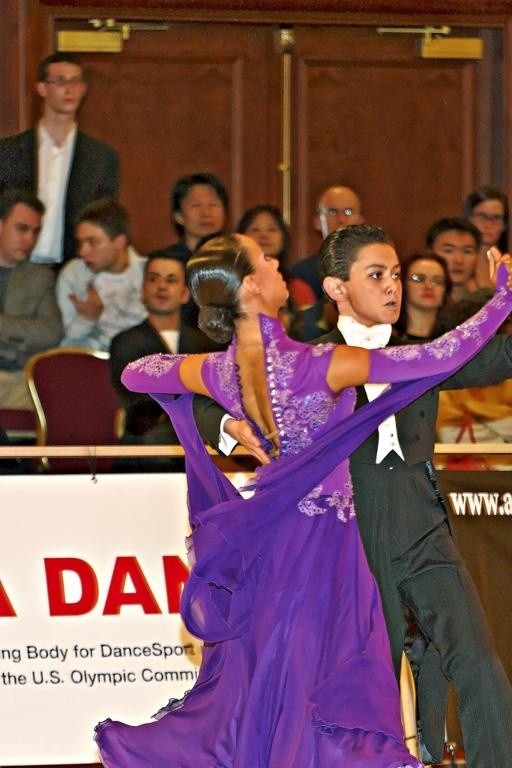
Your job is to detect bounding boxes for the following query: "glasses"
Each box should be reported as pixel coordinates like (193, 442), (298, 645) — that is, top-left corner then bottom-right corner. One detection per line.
(328, 209), (353, 216)
(408, 274), (447, 286)
(473, 214), (504, 225)
(49, 76), (81, 86)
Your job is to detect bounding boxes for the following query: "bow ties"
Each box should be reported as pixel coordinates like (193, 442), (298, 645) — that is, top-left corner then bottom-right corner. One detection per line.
(345, 323), (392, 348)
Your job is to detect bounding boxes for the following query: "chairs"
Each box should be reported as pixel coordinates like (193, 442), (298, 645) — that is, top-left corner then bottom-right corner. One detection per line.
(25, 346), (126, 474)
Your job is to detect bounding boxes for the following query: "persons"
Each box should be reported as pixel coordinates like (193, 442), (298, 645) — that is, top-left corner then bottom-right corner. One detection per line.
(286, 188), (364, 338)
(194, 222), (510, 768)
(429, 216), (490, 307)
(2, 190), (64, 474)
(54, 199), (149, 353)
(405, 254), (453, 339)
(95, 236), (512, 766)
(464, 186), (509, 291)
(237, 205), (322, 337)
(148, 171), (230, 265)
(110, 253), (223, 470)
(0, 54), (119, 268)
